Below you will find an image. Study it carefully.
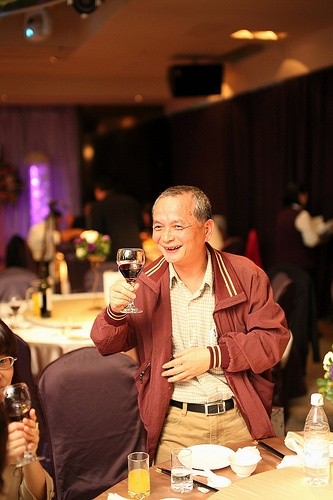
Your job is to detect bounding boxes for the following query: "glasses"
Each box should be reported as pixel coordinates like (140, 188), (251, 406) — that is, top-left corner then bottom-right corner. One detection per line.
(0, 356), (17, 370)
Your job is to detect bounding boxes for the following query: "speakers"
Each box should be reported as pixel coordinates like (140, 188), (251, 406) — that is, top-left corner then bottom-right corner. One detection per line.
(165, 60), (224, 98)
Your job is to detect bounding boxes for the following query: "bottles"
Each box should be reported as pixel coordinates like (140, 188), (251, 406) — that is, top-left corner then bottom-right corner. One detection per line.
(304, 393), (332, 486)
(35, 266), (53, 318)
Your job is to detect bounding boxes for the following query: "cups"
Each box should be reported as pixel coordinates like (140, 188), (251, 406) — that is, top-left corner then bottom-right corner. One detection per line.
(127, 452), (151, 500)
(25, 279), (37, 316)
(171, 449), (194, 493)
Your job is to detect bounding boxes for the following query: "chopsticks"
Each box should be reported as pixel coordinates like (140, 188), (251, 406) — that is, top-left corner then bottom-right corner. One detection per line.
(156, 466), (219, 492)
(254, 440), (285, 459)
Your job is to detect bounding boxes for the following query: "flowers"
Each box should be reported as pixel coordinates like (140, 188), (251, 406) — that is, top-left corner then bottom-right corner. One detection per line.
(75, 229), (111, 259)
(22, 7), (52, 44)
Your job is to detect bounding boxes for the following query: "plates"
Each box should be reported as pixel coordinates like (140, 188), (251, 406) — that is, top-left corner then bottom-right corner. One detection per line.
(177, 444), (235, 470)
(283, 436), (333, 458)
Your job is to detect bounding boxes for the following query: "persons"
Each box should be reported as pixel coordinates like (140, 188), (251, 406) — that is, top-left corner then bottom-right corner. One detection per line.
(91, 186), (293, 466)
(277, 185), (319, 251)
(0, 319), (55, 500)
(5, 233), (44, 279)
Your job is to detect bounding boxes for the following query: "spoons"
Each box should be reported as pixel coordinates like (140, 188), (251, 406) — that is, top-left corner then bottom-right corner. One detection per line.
(203, 467), (231, 487)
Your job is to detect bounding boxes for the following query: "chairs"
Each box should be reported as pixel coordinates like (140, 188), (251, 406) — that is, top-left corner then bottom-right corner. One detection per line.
(15, 318), (148, 500)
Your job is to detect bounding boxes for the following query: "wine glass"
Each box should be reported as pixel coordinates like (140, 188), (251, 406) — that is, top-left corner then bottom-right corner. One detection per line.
(117, 248), (146, 314)
(8, 294), (21, 328)
(0, 383), (45, 468)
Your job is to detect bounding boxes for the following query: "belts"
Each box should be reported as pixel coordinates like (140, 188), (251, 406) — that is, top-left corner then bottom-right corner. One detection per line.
(169, 399), (234, 415)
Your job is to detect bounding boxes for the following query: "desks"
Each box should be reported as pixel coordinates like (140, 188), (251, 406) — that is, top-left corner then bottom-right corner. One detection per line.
(0, 291), (139, 388)
(92, 436), (298, 500)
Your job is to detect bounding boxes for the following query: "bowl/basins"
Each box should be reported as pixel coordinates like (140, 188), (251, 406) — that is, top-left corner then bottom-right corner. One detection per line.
(228, 453), (258, 477)
